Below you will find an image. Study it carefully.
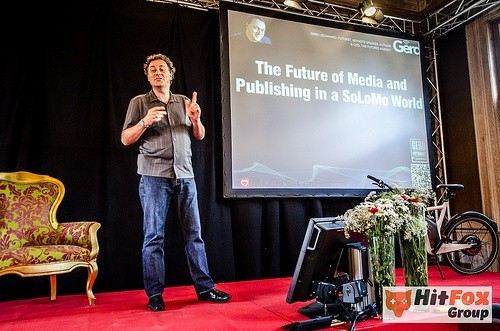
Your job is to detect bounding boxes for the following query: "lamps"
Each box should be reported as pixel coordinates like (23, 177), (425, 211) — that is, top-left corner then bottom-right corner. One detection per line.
(360, 0), (378, 17)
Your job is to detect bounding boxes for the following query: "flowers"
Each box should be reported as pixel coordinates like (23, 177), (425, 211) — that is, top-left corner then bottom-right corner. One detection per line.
(381, 186), (436, 286)
(344, 190), (396, 286)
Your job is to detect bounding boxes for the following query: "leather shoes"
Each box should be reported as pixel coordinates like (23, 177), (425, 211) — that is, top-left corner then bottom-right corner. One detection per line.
(148, 295), (165, 310)
(198, 289), (232, 303)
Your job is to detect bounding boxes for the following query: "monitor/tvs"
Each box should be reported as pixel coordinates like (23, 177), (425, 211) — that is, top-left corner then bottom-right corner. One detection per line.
(286, 216), (369, 304)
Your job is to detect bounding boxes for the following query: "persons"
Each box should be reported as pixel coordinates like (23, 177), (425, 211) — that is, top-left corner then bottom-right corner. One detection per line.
(121, 54), (231, 312)
(230, 14), (271, 43)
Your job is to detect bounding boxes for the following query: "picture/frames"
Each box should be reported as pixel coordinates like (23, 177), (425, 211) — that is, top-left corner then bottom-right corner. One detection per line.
(367, 236), (397, 319)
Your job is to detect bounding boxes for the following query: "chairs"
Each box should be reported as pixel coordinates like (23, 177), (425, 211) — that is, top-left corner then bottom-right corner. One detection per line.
(0, 171), (101, 305)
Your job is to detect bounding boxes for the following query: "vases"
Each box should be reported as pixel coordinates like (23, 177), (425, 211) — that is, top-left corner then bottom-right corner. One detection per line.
(399, 229), (427, 286)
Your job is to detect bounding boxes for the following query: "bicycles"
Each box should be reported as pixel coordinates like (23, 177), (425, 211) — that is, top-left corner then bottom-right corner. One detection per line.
(366, 174), (500, 280)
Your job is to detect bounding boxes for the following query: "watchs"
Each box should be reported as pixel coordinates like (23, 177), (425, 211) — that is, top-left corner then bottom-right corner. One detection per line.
(140, 120), (148, 128)
